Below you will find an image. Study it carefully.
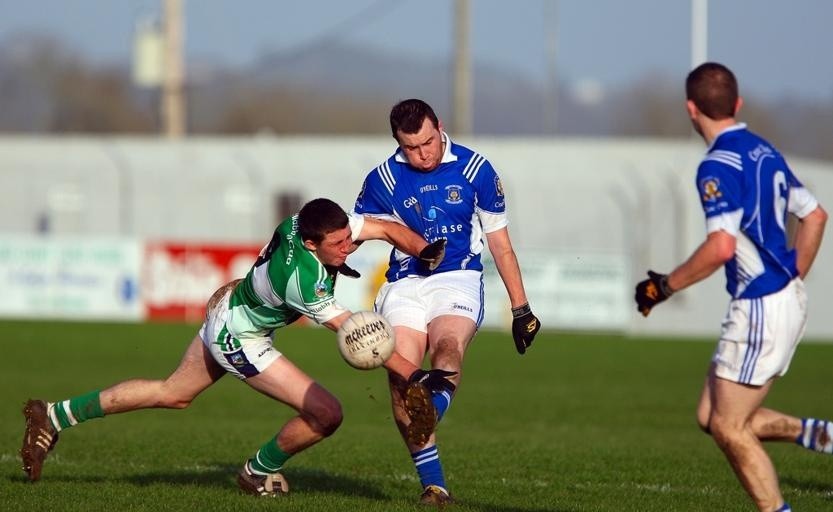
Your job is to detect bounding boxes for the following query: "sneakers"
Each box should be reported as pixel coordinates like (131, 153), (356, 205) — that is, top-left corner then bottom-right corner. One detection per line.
(399, 379), (438, 451)
(419, 484), (453, 507)
(235, 456), (288, 497)
(17, 397), (63, 481)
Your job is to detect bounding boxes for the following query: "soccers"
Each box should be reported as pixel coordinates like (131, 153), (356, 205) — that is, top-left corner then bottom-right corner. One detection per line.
(337, 311), (395, 370)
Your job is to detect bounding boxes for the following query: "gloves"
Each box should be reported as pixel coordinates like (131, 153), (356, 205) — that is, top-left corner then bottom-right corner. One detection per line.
(510, 301), (545, 355)
(416, 237), (448, 276)
(405, 367), (460, 397)
(634, 268), (671, 319)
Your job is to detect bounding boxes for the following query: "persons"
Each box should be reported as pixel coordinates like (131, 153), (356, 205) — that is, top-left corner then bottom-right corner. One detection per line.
(633, 61), (833, 512)
(18, 197), (459, 499)
(343, 97), (543, 507)
